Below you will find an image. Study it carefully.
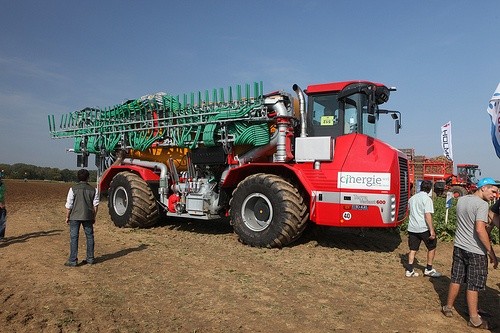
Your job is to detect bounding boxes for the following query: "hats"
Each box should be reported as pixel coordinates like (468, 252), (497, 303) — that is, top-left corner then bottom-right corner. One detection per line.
(476, 176), (500, 189)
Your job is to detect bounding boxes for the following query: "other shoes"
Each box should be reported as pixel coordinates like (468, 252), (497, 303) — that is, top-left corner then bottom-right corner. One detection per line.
(0, 238), (7, 242)
(82, 258), (94, 263)
(467, 318), (497, 330)
(442, 305), (453, 317)
(64, 260), (77, 266)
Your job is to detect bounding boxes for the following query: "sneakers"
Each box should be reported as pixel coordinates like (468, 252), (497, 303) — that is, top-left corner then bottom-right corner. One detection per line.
(424, 267), (441, 277)
(405, 269), (419, 277)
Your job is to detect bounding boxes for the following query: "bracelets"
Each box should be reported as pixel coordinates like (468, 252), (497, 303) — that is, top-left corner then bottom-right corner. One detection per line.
(65, 216), (69, 218)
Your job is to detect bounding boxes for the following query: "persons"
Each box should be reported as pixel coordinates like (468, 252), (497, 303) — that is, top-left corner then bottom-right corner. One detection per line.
(63, 169), (99, 267)
(442, 177), (500, 329)
(489, 197), (500, 229)
(0, 170), (7, 242)
(406, 180), (440, 277)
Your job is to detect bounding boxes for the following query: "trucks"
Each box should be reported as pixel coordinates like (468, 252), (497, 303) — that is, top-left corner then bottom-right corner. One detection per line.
(418, 156), (481, 199)
(97, 80), (415, 251)
